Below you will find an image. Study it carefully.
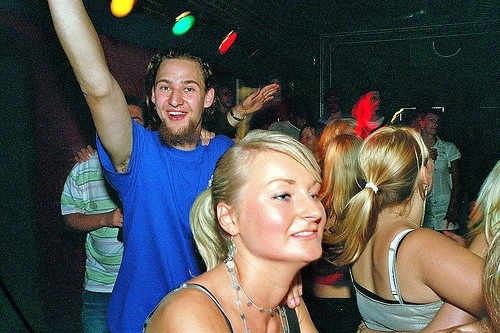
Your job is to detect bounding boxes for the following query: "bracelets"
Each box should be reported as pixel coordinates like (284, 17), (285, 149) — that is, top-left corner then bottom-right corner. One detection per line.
(230, 107), (246, 121)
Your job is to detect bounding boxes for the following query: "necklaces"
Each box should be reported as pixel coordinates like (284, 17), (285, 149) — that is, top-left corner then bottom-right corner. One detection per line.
(224, 256), (289, 332)
(389, 212), (420, 229)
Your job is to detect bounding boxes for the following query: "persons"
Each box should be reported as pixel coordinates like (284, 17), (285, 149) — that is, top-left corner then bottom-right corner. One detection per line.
(31, 27), (113, 333)
(211, 82), (280, 136)
(252, 85), (500, 332)
(46, 0), (303, 333)
(209, 85), (235, 131)
(142, 128), (326, 332)
(58, 93), (215, 333)
(73, 145), (98, 166)
